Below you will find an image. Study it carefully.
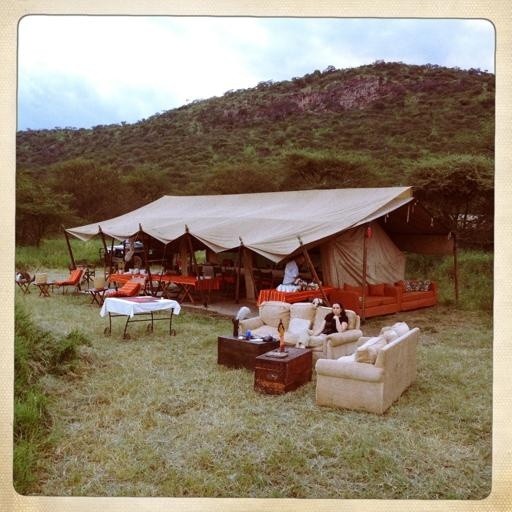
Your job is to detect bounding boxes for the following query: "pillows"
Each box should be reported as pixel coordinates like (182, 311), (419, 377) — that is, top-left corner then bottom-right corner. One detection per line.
(287, 302), (316, 327)
(258, 301), (291, 327)
(355, 321), (410, 363)
(312, 306), (356, 338)
(395, 279), (432, 293)
(344, 282), (386, 295)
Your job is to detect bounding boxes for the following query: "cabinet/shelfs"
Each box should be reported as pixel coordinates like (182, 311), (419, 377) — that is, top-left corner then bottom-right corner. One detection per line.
(217, 333), (280, 374)
(252, 346), (313, 394)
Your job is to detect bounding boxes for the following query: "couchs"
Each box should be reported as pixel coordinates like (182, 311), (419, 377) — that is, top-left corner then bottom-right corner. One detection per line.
(238, 299), (362, 376)
(313, 321), (421, 416)
(328, 281), (402, 322)
(395, 278), (437, 314)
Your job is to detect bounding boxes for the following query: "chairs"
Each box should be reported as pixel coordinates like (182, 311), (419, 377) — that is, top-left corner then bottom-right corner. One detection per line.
(105, 276), (146, 297)
(54, 266), (88, 296)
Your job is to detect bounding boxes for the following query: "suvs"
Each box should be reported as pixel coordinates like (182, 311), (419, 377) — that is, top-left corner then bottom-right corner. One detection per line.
(105, 239), (173, 267)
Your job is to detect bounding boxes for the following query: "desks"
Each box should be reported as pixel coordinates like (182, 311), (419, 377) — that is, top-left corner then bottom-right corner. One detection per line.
(107, 262), (335, 307)
(31, 280), (56, 297)
(85, 287), (108, 305)
(98, 295), (182, 342)
(16, 279), (35, 295)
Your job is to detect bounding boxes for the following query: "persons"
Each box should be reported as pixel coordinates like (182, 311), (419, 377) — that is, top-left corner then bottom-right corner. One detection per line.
(282, 255), (305, 284)
(302, 302), (348, 350)
